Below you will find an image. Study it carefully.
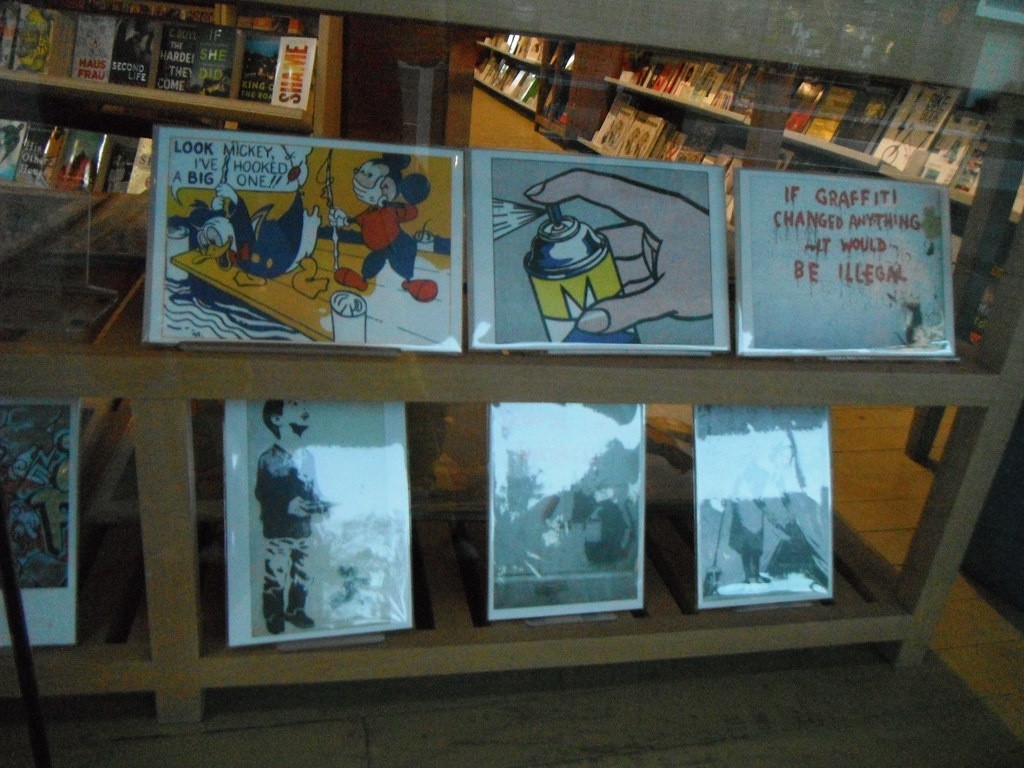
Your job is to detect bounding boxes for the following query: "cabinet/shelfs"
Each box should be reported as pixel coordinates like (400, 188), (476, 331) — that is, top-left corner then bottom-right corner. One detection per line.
(0, 0), (1024, 731)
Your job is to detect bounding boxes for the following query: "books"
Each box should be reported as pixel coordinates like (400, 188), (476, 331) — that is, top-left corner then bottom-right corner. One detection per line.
(1, 117), (154, 194)
(787, 70), (1024, 225)
(474, 55), (569, 125)
(621, 50), (759, 117)
(594, 92), (749, 226)
(1, 4), (319, 114)
(485, 31), (572, 68)
